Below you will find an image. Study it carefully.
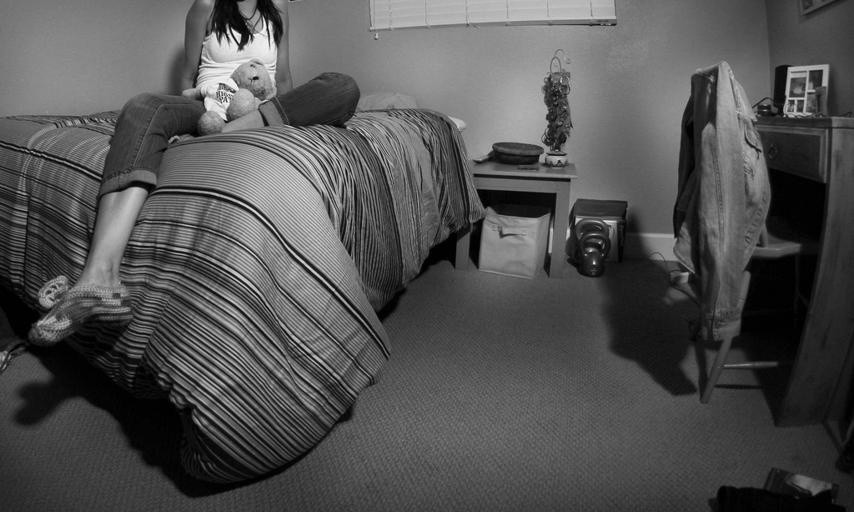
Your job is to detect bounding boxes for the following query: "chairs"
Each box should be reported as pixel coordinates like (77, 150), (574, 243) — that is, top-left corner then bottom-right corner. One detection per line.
(667, 57), (826, 410)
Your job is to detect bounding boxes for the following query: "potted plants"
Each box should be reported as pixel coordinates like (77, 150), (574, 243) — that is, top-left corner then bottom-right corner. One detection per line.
(534, 47), (577, 169)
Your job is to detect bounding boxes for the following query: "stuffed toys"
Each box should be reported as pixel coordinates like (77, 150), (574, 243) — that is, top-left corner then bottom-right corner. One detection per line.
(181, 58), (274, 135)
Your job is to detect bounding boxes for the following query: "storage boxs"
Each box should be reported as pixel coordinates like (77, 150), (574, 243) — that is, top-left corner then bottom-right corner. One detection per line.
(472, 195), (552, 282)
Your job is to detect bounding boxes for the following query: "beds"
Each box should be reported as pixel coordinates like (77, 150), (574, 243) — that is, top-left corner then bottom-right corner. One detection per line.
(0, 84), (490, 496)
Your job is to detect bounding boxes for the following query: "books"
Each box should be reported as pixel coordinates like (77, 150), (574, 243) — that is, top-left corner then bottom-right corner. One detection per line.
(762, 467), (840, 504)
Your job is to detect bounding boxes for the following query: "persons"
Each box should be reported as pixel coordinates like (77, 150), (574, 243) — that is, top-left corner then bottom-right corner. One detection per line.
(28, 0), (360, 347)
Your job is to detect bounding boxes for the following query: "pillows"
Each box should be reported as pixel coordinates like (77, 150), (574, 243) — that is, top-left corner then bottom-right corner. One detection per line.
(350, 89), (421, 116)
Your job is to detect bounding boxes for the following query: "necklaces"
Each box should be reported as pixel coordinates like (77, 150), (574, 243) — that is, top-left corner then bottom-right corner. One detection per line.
(240, 6), (257, 26)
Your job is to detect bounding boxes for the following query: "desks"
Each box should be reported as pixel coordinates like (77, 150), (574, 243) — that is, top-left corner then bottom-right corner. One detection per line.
(747, 102), (854, 479)
(444, 155), (580, 282)
